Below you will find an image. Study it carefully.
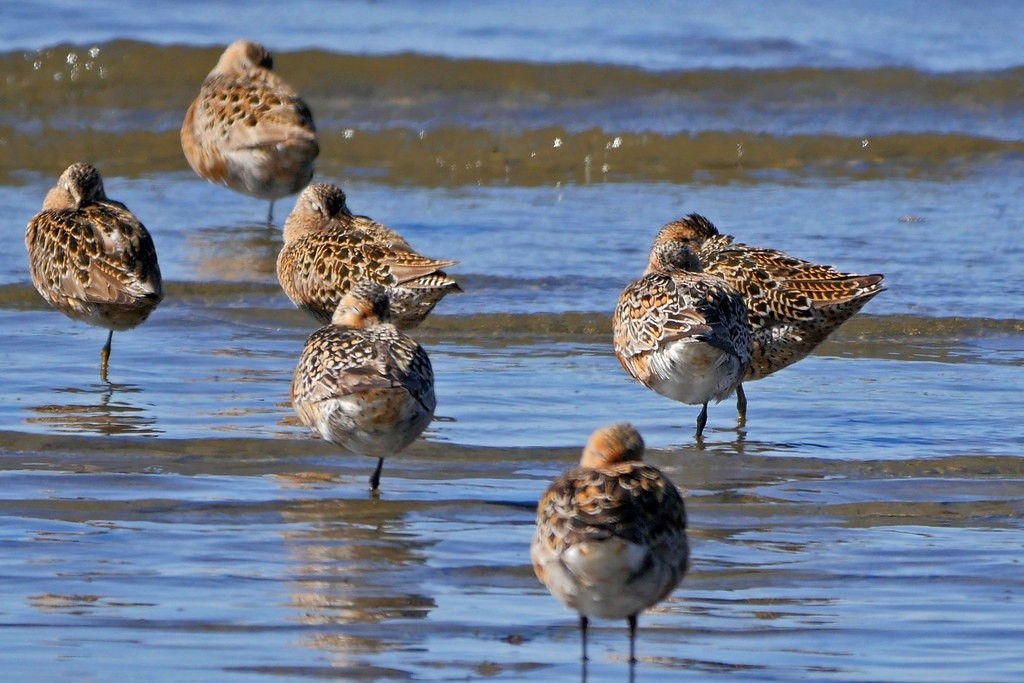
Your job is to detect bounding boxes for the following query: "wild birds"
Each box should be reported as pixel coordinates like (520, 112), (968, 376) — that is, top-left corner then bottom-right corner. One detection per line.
(527, 420), (691, 660)
(179, 38), (322, 222)
(277, 179), (465, 489)
(23, 161), (164, 382)
(611, 212), (888, 434)
